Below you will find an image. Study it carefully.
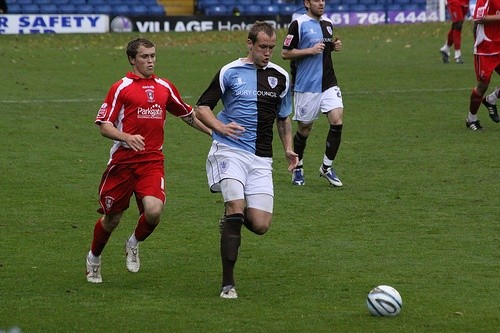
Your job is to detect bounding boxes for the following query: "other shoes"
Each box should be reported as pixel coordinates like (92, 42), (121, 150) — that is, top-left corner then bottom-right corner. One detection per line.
(440, 47), (450, 63)
(455, 56), (464, 64)
(219, 209), (225, 233)
(220, 287), (238, 299)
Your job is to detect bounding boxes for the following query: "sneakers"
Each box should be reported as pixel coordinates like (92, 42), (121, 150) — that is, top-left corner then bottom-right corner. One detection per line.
(482, 96), (500, 123)
(125, 240), (140, 272)
(86, 258), (103, 283)
(466, 119), (482, 131)
(319, 164), (343, 187)
(292, 167), (305, 186)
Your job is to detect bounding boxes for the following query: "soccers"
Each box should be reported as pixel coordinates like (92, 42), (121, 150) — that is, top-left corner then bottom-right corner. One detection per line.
(366, 285), (402, 317)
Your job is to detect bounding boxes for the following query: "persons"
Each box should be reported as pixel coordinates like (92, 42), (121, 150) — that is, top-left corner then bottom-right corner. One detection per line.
(86, 37), (211, 284)
(465, 0), (500, 132)
(194, 21), (300, 299)
(281, 0), (344, 188)
(440, 0), (469, 63)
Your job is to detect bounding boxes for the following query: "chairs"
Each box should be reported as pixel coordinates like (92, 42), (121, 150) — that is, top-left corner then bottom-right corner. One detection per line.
(2, 0), (427, 14)
